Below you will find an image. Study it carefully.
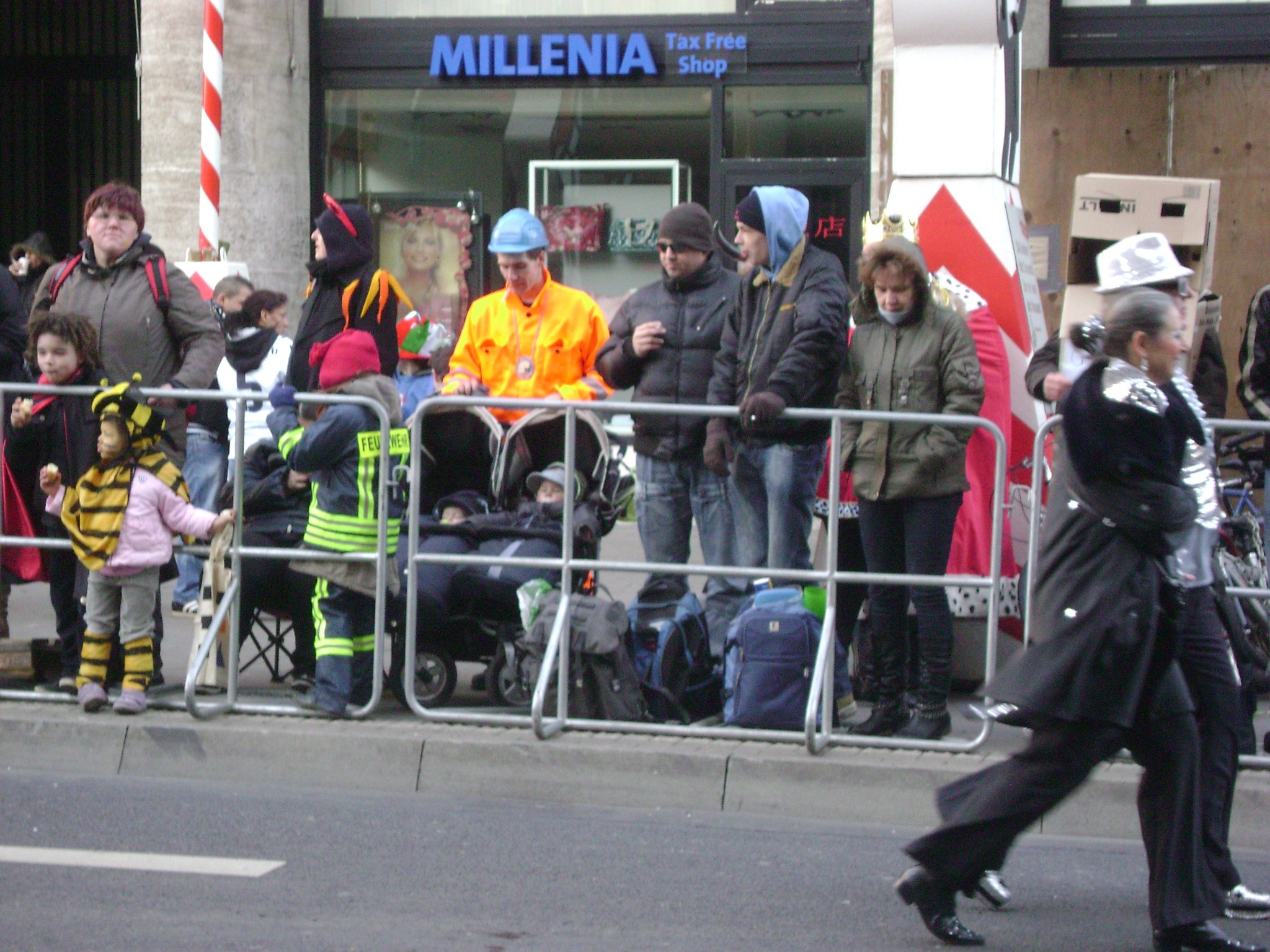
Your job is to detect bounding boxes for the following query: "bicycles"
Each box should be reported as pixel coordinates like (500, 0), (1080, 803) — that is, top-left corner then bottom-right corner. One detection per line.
(1223, 432), (1270, 679)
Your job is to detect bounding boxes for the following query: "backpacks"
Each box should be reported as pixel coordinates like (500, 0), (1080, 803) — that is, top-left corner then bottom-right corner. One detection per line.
(520, 579), (841, 730)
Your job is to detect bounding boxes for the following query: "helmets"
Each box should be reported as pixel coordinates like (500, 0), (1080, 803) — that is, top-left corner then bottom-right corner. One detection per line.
(487, 208), (551, 253)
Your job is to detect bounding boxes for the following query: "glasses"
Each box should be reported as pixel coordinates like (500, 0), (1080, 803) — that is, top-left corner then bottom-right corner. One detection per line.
(657, 242), (688, 253)
(1154, 279), (1190, 298)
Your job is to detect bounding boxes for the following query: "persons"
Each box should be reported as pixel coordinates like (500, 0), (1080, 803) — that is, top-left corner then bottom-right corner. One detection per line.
(393, 222), (444, 316)
(836, 235), (984, 742)
(0, 179), (455, 721)
(592, 203), (740, 673)
(891, 234), (1270, 952)
(441, 208), (615, 688)
(461, 461), (599, 543)
(703, 184), (870, 726)
(432, 490), (490, 524)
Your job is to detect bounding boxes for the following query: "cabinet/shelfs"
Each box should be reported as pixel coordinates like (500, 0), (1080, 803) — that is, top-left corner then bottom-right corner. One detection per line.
(522, 149), (691, 331)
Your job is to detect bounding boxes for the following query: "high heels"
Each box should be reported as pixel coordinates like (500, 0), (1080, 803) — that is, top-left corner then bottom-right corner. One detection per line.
(894, 863), (985, 946)
(1152, 918), (1267, 952)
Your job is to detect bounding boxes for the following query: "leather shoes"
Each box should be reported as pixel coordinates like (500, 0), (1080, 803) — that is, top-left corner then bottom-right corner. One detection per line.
(848, 708), (912, 735)
(893, 710), (952, 739)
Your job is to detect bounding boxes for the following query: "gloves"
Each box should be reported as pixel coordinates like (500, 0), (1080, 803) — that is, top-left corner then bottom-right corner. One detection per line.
(739, 392), (786, 432)
(703, 420), (734, 476)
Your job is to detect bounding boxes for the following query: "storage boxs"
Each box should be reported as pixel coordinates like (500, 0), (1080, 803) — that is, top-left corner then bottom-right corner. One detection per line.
(1059, 172), (1221, 409)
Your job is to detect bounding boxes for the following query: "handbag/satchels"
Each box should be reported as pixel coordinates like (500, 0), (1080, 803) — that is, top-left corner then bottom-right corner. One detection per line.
(189, 524), (236, 693)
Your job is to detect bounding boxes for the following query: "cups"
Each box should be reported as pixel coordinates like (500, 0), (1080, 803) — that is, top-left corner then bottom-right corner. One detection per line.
(804, 586), (827, 620)
(753, 577), (774, 593)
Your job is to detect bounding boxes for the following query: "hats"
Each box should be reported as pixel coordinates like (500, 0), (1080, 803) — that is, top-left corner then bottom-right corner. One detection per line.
(1094, 232), (1196, 293)
(308, 331), (382, 386)
(526, 467), (566, 492)
(735, 192), (765, 234)
(659, 202), (715, 252)
(395, 314), (438, 359)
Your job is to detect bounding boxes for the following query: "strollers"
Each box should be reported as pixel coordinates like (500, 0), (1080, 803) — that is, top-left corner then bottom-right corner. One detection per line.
(382, 393), (639, 713)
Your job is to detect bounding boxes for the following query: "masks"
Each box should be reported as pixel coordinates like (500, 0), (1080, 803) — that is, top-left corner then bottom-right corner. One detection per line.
(876, 304), (914, 324)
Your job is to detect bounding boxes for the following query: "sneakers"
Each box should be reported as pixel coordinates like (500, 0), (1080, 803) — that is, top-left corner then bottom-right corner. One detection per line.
(112, 689), (146, 713)
(77, 684), (109, 710)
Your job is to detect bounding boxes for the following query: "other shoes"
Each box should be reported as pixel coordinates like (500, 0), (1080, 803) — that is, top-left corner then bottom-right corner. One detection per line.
(59, 676), (78, 692)
(1222, 884), (1270, 919)
(291, 676), (313, 691)
(34, 682), (59, 695)
(173, 599), (198, 615)
(976, 870), (1012, 907)
(293, 692), (339, 720)
